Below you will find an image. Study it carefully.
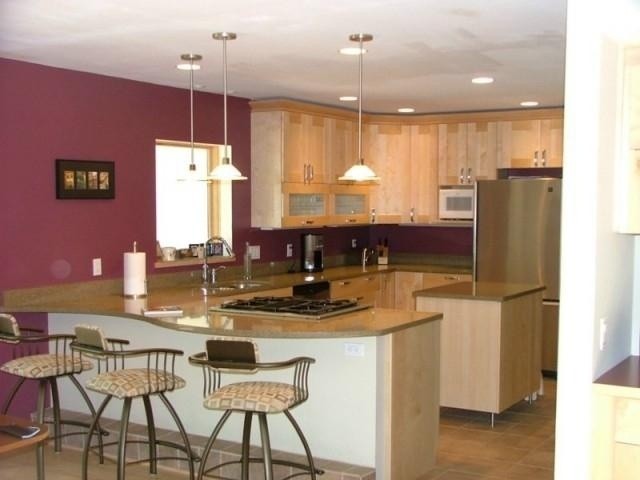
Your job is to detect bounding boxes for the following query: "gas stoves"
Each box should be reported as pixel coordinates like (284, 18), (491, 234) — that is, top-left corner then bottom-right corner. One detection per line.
(208, 296), (370, 320)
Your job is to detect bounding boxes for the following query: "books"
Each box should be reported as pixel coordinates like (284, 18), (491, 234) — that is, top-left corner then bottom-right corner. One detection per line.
(141, 306), (183, 316)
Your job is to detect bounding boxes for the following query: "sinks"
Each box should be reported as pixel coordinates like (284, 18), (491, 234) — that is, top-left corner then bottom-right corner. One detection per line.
(220, 281), (272, 292)
(183, 285), (238, 297)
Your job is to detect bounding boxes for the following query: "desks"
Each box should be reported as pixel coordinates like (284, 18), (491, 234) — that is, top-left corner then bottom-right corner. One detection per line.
(0, 412), (52, 480)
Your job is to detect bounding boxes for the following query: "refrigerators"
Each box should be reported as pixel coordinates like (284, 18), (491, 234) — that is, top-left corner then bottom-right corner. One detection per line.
(474, 176), (556, 374)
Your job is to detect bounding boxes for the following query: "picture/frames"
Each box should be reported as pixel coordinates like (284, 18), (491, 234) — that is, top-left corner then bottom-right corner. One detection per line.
(54, 157), (115, 202)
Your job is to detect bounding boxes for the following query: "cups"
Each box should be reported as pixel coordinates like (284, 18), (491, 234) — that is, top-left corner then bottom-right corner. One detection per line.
(162, 247), (177, 262)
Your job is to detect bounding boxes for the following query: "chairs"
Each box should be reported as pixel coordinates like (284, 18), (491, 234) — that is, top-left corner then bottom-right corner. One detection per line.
(0, 311), (109, 479)
(68, 325), (202, 479)
(187, 336), (328, 479)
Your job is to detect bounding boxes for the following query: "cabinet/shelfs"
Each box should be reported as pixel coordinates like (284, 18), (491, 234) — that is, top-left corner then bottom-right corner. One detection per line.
(326, 103), (369, 229)
(399, 114), (440, 226)
(367, 115), (402, 228)
(437, 111), (495, 188)
(409, 280), (548, 428)
(250, 99), (327, 231)
(328, 270), (472, 307)
(497, 108), (563, 168)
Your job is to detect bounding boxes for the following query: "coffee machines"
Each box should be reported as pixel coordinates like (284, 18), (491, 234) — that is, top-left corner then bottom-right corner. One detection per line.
(301, 232), (325, 273)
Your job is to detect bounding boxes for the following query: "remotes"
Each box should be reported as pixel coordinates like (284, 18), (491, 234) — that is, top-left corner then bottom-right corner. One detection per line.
(141, 306), (183, 318)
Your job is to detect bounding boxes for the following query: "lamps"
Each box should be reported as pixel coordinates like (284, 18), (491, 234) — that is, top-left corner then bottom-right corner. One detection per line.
(178, 53), (207, 185)
(210, 32), (251, 184)
(337, 32), (381, 184)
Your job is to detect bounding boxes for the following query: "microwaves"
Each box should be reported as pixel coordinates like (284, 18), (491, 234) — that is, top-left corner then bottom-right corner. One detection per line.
(438, 187), (474, 220)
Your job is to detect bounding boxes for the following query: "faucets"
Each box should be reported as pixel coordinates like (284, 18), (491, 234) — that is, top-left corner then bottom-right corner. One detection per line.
(361, 248), (374, 272)
(201, 236), (234, 284)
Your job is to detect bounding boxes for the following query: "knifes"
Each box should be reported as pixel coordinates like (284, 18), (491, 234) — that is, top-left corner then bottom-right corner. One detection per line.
(377, 236), (388, 247)
